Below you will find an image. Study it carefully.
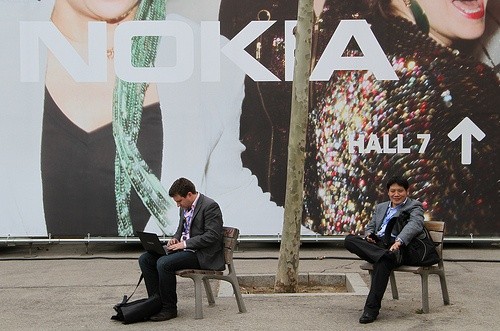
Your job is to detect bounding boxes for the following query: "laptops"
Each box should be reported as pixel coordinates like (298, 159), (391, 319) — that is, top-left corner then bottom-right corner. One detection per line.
(136, 231), (184, 255)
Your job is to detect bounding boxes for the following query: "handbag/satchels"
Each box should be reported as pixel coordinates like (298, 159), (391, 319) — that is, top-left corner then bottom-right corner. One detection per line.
(111, 294), (162, 325)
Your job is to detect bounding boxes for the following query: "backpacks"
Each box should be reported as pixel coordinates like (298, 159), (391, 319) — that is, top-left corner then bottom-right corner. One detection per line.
(391, 211), (440, 267)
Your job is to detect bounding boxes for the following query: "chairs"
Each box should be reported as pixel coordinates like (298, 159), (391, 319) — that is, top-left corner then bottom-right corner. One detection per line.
(175, 227), (247, 320)
(361, 221), (449, 314)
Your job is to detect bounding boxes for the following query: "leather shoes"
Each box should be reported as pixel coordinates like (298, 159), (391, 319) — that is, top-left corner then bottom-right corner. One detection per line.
(150, 308), (177, 322)
(359, 312), (376, 323)
(385, 250), (402, 263)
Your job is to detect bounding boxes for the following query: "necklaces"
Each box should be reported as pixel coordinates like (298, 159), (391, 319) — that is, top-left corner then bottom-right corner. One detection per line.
(106, 48), (114, 58)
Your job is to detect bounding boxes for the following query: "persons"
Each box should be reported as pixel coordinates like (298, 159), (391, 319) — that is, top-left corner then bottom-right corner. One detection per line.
(344, 176), (426, 324)
(0, 0), (500, 237)
(139, 178), (225, 322)
